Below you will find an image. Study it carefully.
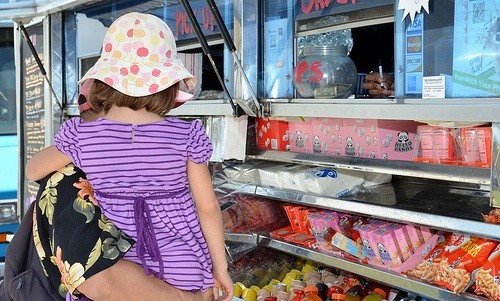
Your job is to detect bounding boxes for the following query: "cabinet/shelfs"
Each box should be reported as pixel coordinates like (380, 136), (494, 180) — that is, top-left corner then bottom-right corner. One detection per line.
(210, 124), (500, 300)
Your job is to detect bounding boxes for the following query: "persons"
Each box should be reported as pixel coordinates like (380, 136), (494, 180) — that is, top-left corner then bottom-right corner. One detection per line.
(0, 78), (214, 301)
(25, 11), (234, 301)
(362, 71), (393, 97)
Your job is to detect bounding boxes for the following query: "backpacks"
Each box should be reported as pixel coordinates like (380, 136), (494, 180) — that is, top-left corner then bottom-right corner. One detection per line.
(0, 172), (73, 301)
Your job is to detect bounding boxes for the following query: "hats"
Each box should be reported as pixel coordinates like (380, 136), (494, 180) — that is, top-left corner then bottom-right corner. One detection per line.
(76, 12), (196, 97)
(78, 78), (194, 113)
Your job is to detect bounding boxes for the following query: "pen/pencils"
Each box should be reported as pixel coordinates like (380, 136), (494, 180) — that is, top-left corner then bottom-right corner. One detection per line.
(379, 66), (384, 90)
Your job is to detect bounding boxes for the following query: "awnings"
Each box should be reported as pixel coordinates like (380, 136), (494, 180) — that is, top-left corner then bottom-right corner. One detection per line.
(11, 0), (261, 114)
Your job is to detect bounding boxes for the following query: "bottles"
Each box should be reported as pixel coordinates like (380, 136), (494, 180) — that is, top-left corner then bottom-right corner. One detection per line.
(227, 250), (432, 301)
